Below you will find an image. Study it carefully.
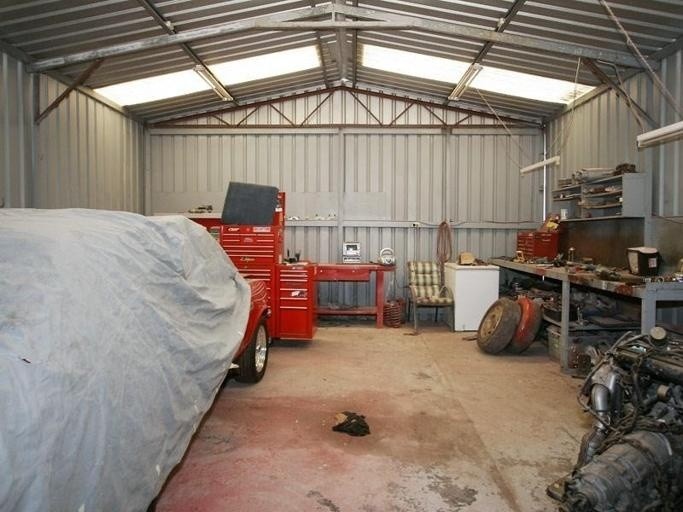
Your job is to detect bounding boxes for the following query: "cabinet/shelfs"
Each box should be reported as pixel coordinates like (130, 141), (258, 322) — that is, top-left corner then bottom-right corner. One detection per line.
(551, 173), (653, 222)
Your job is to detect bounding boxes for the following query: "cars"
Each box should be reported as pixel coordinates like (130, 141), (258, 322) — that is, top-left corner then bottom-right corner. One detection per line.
(230, 279), (271, 384)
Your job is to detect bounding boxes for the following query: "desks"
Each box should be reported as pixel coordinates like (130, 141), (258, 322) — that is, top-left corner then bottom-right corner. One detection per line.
(488, 258), (683, 370)
(316, 263), (396, 329)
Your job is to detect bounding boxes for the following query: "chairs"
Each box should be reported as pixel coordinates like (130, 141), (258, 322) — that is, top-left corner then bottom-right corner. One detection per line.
(405, 260), (454, 331)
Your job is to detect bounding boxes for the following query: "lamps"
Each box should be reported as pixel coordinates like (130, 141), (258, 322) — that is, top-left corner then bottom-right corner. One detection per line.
(447, 63), (483, 101)
(519, 155), (560, 174)
(193, 65), (234, 102)
(637, 120), (683, 149)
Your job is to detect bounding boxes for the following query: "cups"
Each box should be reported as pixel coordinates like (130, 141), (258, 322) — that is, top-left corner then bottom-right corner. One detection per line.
(561, 209), (568, 220)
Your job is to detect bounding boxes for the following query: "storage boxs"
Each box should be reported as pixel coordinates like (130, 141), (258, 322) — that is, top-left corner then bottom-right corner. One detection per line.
(547, 328), (614, 364)
